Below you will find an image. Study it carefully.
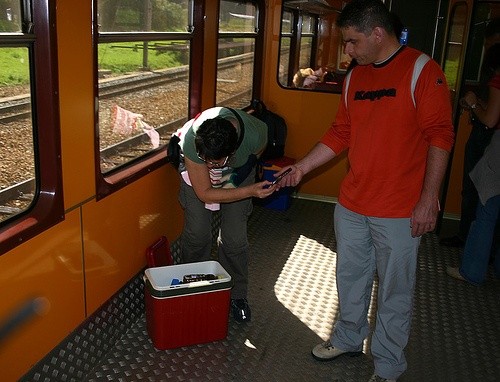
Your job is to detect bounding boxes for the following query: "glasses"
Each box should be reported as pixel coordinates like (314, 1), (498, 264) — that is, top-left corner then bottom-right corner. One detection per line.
(196, 151), (229, 168)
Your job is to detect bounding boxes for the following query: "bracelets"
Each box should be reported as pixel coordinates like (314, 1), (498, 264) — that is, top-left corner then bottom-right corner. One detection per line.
(472, 104), (478, 108)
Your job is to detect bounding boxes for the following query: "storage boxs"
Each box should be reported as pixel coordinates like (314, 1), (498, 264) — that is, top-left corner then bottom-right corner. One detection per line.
(145, 261), (234, 350)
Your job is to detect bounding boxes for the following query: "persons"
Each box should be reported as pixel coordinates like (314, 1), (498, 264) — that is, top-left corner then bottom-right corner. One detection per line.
(439, 17), (499, 246)
(274, 0), (455, 382)
(446, 44), (500, 283)
(179, 106), (278, 322)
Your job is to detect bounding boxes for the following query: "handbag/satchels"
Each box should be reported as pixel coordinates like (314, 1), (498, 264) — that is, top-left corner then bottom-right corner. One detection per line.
(167, 136), (272, 189)
(251, 96), (287, 159)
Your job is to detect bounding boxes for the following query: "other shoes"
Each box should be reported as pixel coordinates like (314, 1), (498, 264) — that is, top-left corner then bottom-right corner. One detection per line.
(447, 265), (481, 285)
(369, 374), (397, 382)
(231, 297), (251, 321)
(311, 339), (365, 360)
